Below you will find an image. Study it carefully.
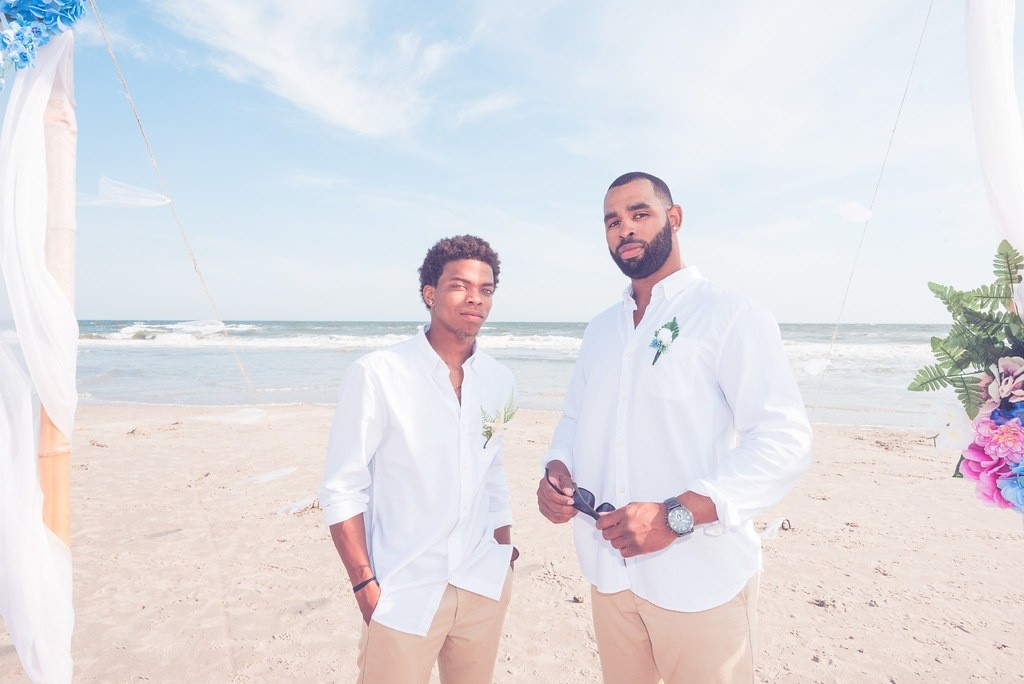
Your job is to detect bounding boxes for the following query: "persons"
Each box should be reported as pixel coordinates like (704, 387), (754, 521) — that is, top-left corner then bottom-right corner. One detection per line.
(320, 234), (519, 684)
(537, 171), (812, 683)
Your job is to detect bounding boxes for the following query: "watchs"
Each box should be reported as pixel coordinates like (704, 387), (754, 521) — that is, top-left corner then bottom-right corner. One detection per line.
(665, 496), (695, 537)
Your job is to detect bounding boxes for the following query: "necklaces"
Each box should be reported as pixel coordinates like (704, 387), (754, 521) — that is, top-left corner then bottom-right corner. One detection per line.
(453, 385), (461, 392)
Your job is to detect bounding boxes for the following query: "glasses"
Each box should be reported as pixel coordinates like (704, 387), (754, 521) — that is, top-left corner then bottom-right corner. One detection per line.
(545, 467), (615, 521)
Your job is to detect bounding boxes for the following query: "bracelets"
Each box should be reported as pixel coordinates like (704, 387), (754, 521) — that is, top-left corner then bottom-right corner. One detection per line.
(353, 576), (376, 593)
